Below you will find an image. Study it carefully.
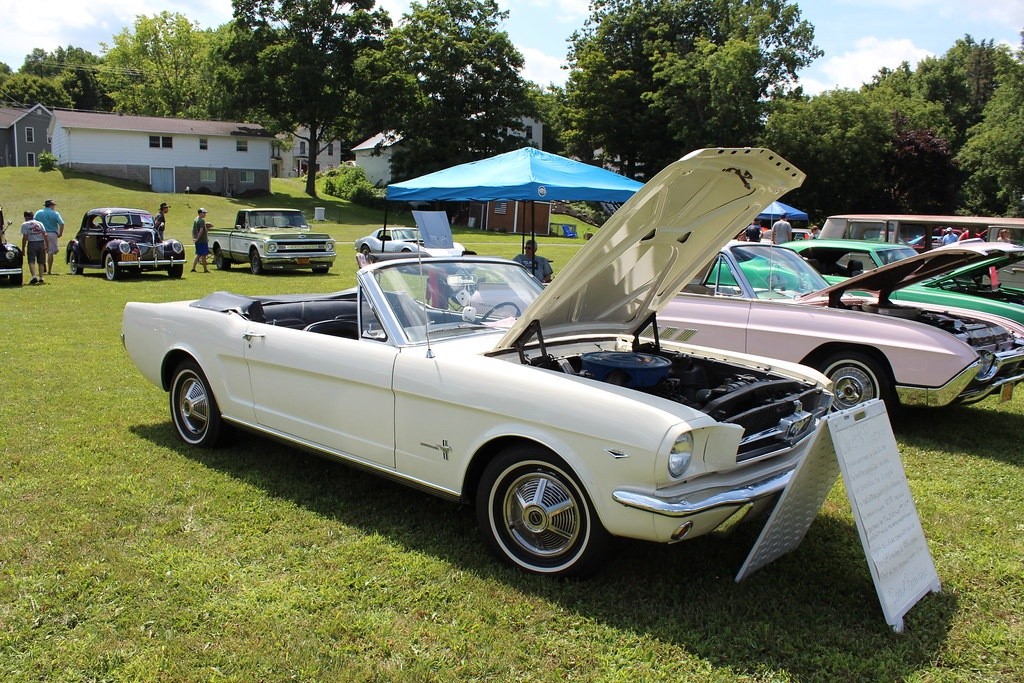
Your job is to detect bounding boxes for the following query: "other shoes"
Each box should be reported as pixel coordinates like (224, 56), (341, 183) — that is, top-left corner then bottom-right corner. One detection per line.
(204, 270), (210, 273)
(29, 278), (37, 284)
(39, 279), (44, 283)
(191, 269), (196, 272)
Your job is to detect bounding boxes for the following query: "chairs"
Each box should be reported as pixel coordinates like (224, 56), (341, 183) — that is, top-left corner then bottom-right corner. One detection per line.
(838, 260), (864, 277)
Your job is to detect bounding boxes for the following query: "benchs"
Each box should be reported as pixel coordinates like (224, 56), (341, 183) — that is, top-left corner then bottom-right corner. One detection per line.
(264, 301), (375, 331)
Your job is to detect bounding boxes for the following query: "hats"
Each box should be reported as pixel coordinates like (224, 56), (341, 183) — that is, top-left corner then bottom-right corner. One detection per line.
(947, 227), (952, 231)
(198, 208), (208, 214)
(43, 200), (56, 206)
(753, 218), (759, 221)
(158, 203), (169, 210)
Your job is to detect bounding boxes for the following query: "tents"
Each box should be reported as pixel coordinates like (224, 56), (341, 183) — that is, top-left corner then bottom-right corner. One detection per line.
(380, 147), (648, 262)
(756, 201), (811, 225)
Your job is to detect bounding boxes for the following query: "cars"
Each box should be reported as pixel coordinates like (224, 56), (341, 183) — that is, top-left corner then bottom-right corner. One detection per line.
(464, 237), (1024, 419)
(120, 148), (834, 583)
(761, 214), (1023, 291)
(355, 227), (467, 260)
(708, 239), (1024, 340)
(0, 206), (23, 286)
(66, 208), (187, 282)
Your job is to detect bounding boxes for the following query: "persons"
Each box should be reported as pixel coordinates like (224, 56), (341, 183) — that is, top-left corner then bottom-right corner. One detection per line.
(21, 211), (50, 284)
(446, 251), (478, 308)
(736, 217), (763, 242)
(808, 226), (818, 241)
(154, 203), (171, 242)
(33, 200), (64, 276)
(959, 226), (979, 243)
(190, 208), (214, 273)
(771, 215), (792, 244)
(943, 227), (958, 247)
(997, 229), (1011, 244)
(513, 240), (553, 284)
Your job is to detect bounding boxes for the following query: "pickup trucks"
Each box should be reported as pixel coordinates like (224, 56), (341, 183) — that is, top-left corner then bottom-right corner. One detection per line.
(207, 208), (337, 276)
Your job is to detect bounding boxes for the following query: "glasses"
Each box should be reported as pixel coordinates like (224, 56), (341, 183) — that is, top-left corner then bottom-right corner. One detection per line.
(524, 246), (532, 250)
